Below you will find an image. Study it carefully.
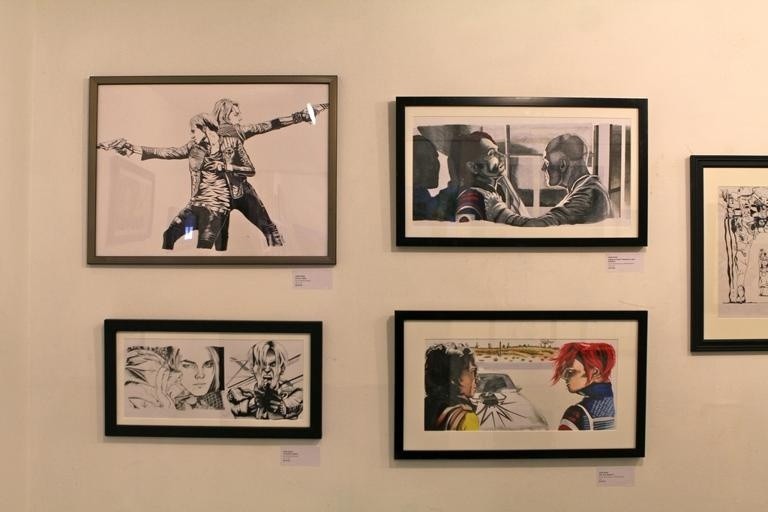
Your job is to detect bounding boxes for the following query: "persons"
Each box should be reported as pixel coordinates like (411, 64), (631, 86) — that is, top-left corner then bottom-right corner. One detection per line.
(443, 130), (534, 223)
(166, 347), (224, 411)
(112, 111), (257, 251)
(226, 339), (303, 420)
(547, 340), (616, 429)
(214, 96), (328, 252)
(423, 340), (481, 431)
(410, 132), (442, 221)
(484, 133), (619, 228)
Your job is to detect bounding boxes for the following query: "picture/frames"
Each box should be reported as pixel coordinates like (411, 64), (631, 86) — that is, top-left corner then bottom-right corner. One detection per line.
(396, 310), (648, 458)
(88, 76), (337, 265)
(691, 155), (767, 351)
(105, 319), (321, 439)
(396, 96), (648, 246)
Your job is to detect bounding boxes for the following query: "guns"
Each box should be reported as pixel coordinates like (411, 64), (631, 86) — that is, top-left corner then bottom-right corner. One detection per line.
(97, 137), (133, 155)
(260, 381), (274, 412)
(303, 102), (328, 122)
